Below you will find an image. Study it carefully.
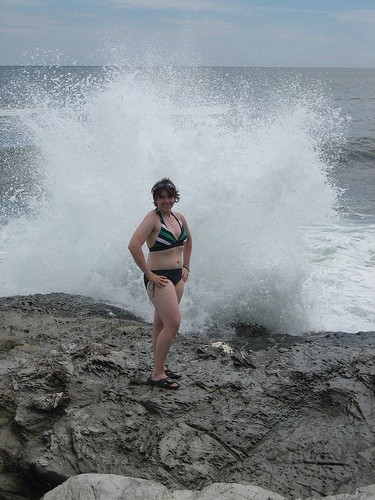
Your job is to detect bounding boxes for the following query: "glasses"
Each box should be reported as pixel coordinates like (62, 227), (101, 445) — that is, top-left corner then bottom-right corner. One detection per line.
(156, 182), (175, 191)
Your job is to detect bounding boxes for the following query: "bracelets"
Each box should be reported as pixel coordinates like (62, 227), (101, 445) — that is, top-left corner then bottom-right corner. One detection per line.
(182, 265), (190, 272)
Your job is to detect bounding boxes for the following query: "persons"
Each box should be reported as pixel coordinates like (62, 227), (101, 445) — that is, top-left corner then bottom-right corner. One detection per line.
(128, 177), (192, 390)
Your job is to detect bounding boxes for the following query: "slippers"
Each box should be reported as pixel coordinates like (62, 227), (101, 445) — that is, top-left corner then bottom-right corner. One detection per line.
(147, 373), (180, 390)
(164, 369), (182, 379)
